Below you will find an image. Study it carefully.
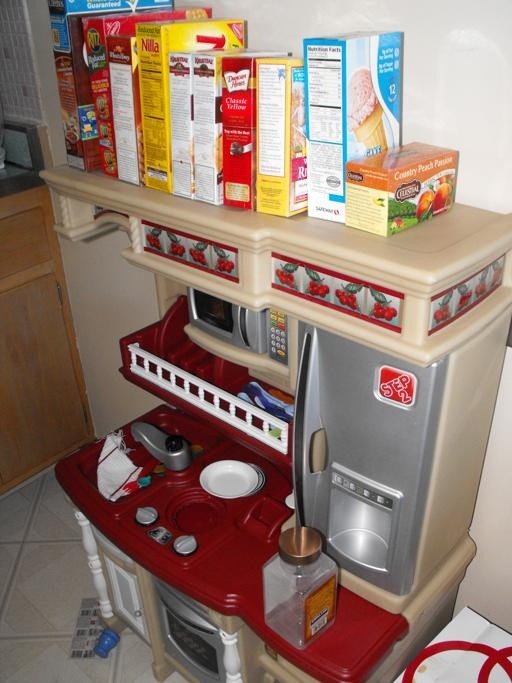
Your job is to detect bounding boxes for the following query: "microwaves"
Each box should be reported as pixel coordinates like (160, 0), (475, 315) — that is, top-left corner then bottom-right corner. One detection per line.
(187, 286), (288, 368)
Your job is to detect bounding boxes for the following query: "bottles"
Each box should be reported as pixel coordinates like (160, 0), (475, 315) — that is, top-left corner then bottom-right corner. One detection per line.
(262, 529), (338, 651)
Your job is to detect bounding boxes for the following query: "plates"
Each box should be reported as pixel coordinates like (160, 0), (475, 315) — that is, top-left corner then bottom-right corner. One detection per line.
(199, 460), (266, 499)
